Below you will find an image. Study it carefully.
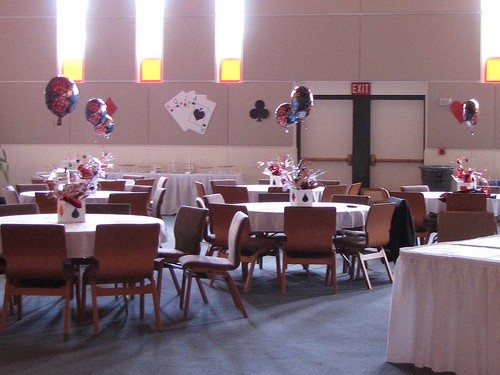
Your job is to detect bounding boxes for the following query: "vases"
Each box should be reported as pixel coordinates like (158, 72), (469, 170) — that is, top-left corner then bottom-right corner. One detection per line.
(57, 200), (87, 223)
(270, 176), (282, 187)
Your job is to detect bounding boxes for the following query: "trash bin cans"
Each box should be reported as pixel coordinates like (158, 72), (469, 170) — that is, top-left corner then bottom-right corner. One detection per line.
(417, 165), (455, 192)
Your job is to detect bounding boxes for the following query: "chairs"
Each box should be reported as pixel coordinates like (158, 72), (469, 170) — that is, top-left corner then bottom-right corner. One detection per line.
(0, 175), (500, 342)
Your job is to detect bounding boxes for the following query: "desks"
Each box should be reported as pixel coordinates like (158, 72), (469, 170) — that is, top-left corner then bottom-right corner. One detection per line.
(238, 183), (325, 204)
(0, 214), (168, 259)
(385, 234), (500, 375)
(486, 194), (500, 216)
(233, 201), (371, 233)
(19, 189), (119, 204)
(421, 191), (447, 214)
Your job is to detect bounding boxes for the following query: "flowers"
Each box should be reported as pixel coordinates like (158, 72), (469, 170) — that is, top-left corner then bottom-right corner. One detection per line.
(255, 153), (295, 191)
(291, 158), (328, 189)
(450, 157), (492, 192)
(39, 151), (117, 209)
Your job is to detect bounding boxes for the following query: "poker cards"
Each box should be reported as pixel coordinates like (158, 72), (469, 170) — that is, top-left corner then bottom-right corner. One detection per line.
(164, 90), (216, 135)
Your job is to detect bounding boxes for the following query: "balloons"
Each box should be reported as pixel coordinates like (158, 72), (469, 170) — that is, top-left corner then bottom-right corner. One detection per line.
(462, 99), (480, 135)
(95, 115), (114, 137)
(290, 85), (314, 122)
(275, 104), (295, 133)
(86, 98), (107, 126)
(45, 76), (79, 126)
